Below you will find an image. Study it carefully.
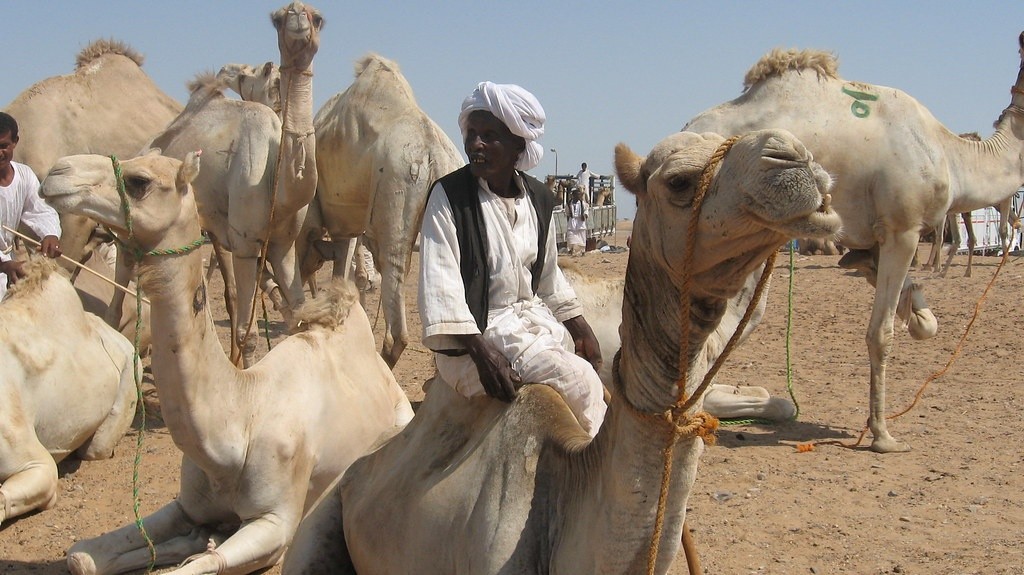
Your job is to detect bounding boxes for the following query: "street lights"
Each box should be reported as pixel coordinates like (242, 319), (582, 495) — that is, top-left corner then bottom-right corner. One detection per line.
(551, 148), (557, 185)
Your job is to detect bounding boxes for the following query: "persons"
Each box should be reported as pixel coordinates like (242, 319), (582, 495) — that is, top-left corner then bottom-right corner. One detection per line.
(565, 162), (605, 256)
(95, 241), (116, 272)
(350, 243), (377, 295)
(418, 81), (608, 440)
(0, 112), (63, 301)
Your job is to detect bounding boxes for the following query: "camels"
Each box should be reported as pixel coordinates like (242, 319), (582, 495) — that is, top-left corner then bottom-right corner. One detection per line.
(673, 30), (1024, 453)
(42, 149), (414, 574)
(0, 252), (143, 525)
(280, 128), (843, 574)
(0, 0), (467, 371)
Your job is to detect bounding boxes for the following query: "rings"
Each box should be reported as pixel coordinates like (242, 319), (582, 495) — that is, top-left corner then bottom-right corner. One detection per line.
(56, 246), (58, 248)
